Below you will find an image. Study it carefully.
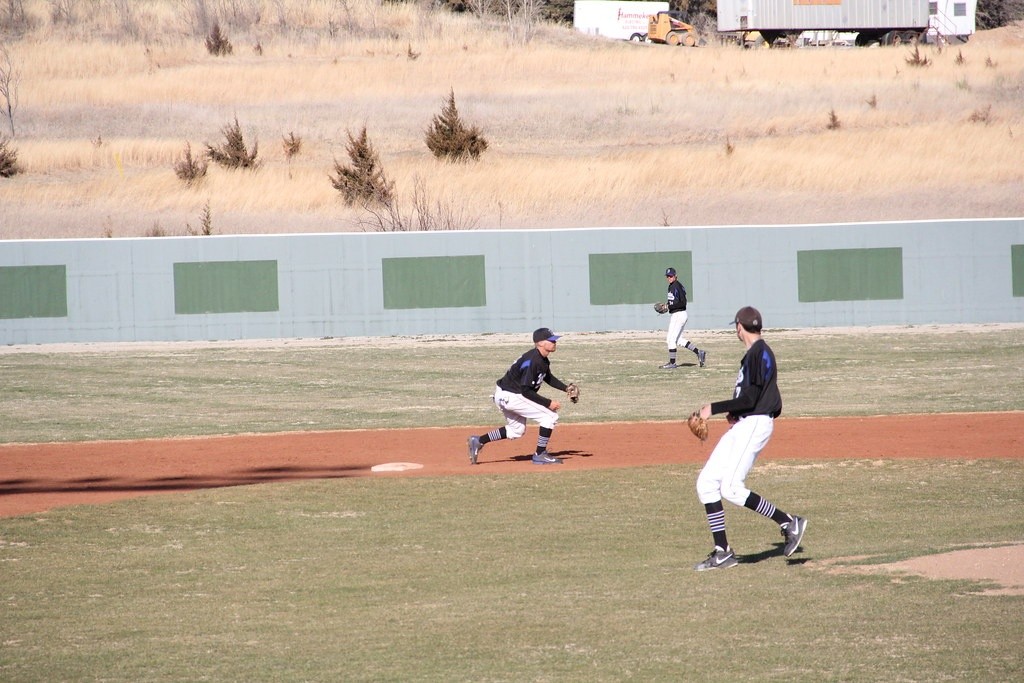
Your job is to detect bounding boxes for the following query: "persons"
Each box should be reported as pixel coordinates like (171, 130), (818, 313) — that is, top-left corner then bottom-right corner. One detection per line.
(468, 328), (580, 465)
(654, 268), (706, 369)
(688, 306), (807, 570)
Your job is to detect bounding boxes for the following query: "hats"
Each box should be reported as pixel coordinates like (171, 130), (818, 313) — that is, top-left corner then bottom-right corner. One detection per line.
(729, 306), (763, 334)
(664, 268), (676, 276)
(533, 328), (561, 343)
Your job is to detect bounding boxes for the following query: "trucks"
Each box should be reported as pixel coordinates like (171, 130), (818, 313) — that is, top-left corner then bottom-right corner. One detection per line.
(572, 0), (671, 44)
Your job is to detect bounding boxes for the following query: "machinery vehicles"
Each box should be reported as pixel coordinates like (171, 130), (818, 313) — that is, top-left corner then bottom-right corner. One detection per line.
(737, 30), (770, 49)
(645, 6), (708, 48)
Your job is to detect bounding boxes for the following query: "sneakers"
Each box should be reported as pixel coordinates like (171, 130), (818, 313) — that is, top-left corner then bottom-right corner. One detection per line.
(659, 362), (677, 369)
(697, 350), (706, 367)
(780, 513), (807, 556)
(692, 546), (738, 571)
(532, 451), (563, 465)
(468, 435), (485, 464)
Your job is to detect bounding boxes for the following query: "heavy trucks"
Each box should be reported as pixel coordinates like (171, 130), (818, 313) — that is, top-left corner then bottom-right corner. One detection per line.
(716, 0), (978, 50)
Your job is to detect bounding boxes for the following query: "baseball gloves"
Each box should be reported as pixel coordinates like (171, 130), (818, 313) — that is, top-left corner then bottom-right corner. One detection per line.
(654, 303), (668, 314)
(568, 383), (579, 403)
(688, 409), (709, 440)
